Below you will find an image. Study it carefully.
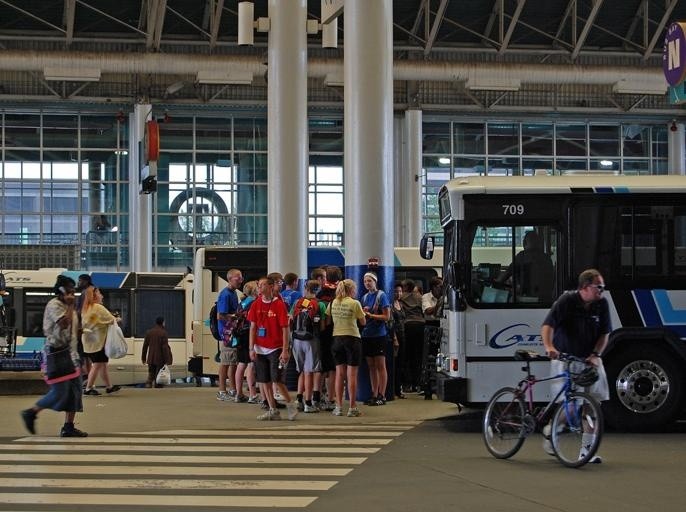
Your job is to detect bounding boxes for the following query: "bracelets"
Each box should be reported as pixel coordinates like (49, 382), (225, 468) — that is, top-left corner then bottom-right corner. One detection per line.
(592, 351), (603, 358)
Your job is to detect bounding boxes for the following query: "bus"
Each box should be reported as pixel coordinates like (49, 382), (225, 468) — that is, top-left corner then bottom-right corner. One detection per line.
(183, 240), (686, 382)
(417, 175), (686, 426)
(0, 267), (204, 387)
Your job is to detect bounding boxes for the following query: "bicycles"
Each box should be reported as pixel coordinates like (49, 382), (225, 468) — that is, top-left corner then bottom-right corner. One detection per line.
(471, 351), (606, 469)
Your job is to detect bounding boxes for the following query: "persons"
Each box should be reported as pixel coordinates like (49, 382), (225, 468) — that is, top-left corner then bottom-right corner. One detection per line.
(81, 286), (123, 396)
(541, 268), (611, 463)
(209, 264), (444, 418)
(76, 275), (94, 390)
(141, 316), (168, 388)
(20, 275), (89, 438)
(494, 231), (555, 302)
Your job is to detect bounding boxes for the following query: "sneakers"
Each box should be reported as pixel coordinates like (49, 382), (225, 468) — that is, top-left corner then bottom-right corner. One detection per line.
(215, 385), (287, 410)
(106, 386), (122, 393)
(59, 426), (88, 437)
(346, 409), (366, 418)
(332, 407), (345, 417)
(294, 390), (337, 414)
(577, 448), (603, 464)
(80, 378), (103, 396)
(21, 408), (38, 435)
(386, 391), (408, 402)
(287, 400), (299, 421)
(363, 393), (387, 407)
(543, 426), (560, 454)
(257, 409), (282, 421)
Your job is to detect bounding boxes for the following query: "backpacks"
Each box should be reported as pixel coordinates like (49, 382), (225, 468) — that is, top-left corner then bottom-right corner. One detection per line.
(209, 301), (223, 342)
(362, 290), (396, 345)
(288, 297), (322, 342)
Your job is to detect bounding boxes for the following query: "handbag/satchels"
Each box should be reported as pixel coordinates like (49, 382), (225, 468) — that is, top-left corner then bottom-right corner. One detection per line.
(40, 344), (82, 385)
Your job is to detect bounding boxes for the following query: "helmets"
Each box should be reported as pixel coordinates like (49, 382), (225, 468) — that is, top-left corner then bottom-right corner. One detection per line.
(572, 367), (600, 387)
